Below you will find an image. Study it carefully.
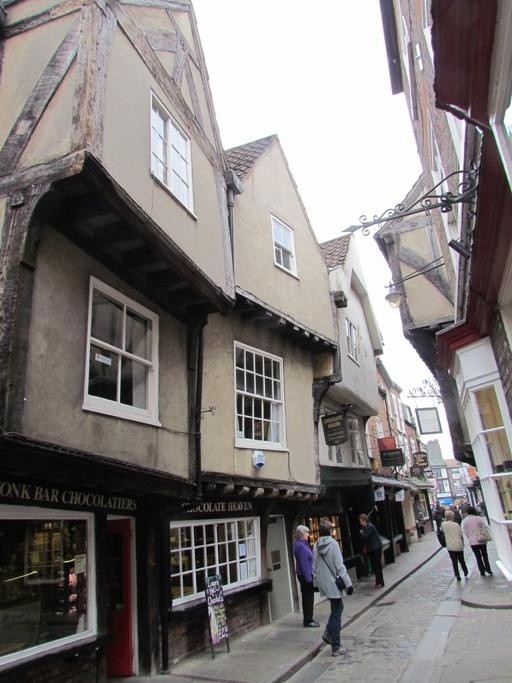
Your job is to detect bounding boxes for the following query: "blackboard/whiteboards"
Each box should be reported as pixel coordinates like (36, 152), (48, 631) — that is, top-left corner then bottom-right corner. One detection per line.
(205, 576), (230, 645)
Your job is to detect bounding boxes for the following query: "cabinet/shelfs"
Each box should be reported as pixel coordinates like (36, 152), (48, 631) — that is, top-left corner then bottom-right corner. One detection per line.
(24, 528), (72, 584)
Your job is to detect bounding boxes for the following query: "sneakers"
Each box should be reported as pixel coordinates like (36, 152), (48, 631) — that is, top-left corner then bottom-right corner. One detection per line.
(332, 645), (348, 656)
(322, 630), (333, 645)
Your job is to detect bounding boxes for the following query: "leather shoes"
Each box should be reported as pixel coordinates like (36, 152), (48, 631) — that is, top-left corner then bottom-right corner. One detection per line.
(480, 568), (493, 576)
(457, 570), (468, 581)
(303, 619), (320, 627)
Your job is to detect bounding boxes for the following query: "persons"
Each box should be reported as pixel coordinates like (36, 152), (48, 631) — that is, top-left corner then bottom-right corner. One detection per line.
(432, 497), (492, 581)
(359, 512), (385, 587)
(311, 523), (354, 657)
(293, 524), (321, 627)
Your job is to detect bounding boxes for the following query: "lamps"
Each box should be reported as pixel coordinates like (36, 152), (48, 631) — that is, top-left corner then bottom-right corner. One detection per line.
(384, 255), (444, 309)
(201, 404), (217, 419)
(253, 450), (265, 469)
(318, 402), (358, 432)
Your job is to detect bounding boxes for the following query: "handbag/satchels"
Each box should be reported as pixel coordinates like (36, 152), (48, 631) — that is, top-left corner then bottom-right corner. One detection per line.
(436, 530), (446, 547)
(335, 575), (346, 591)
(474, 527), (492, 542)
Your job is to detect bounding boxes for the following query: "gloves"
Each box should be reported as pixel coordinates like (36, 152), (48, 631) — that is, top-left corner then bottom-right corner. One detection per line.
(313, 587), (319, 592)
(347, 586), (354, 595)
